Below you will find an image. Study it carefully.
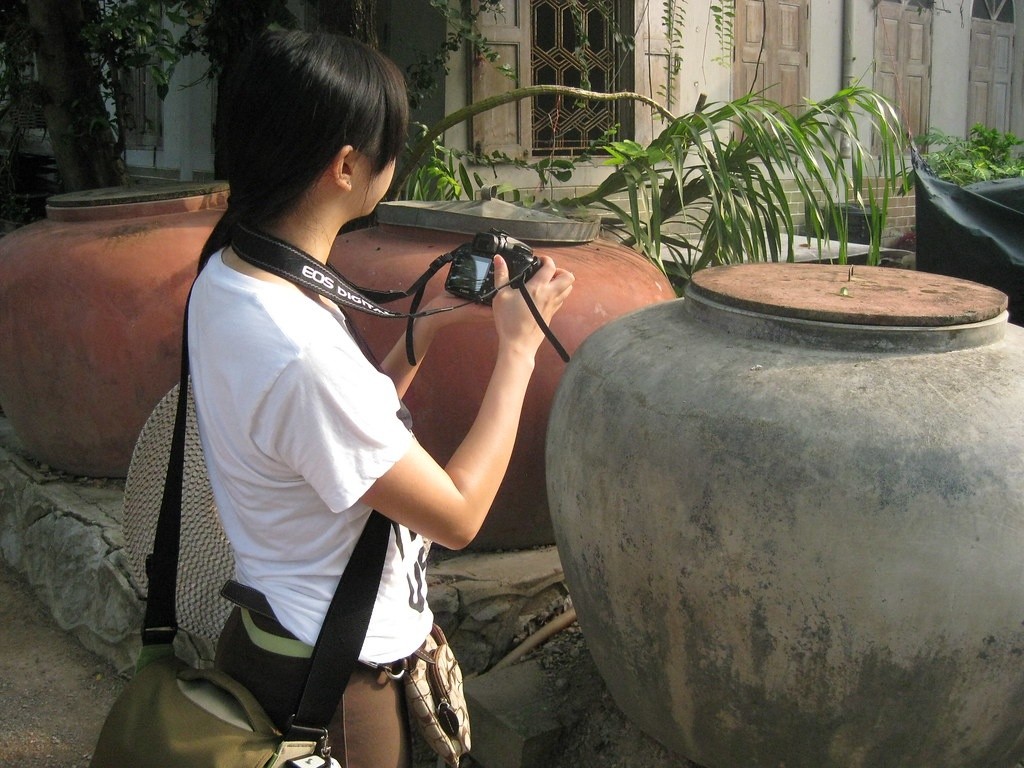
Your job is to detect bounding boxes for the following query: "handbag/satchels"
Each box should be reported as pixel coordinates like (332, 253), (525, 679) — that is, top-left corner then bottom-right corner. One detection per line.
(88, 643), (342, 768)
(405, 623), (472, 768)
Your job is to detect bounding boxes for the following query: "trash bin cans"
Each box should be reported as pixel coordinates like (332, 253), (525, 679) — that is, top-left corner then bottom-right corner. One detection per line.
(820, 203), (888, 247)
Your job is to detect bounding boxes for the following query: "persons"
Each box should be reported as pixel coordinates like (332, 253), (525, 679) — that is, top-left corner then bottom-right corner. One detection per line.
(187, 29), (575, 768)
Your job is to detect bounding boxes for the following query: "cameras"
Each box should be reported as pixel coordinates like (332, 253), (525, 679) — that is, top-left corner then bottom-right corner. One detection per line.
(444, 227), (545, 306)
